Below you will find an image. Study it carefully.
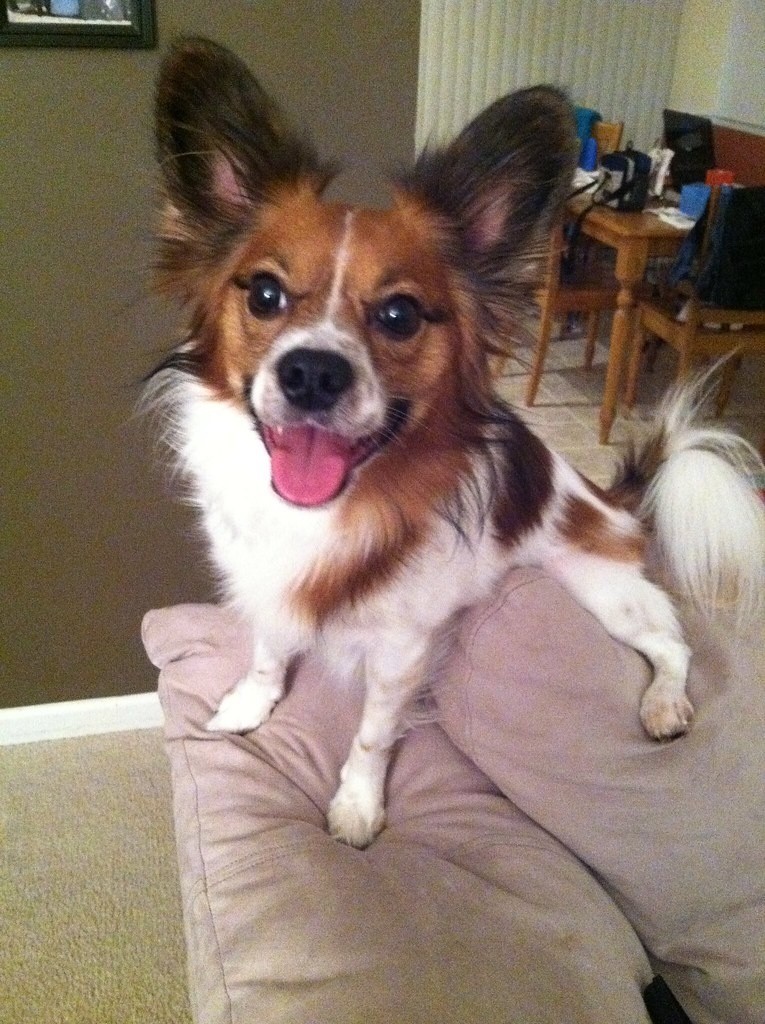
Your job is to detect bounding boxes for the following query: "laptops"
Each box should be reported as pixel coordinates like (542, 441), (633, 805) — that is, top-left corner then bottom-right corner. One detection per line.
(664, 109), (717, 195)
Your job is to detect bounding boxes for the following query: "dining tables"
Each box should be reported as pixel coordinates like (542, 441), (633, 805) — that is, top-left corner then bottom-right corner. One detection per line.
(524, 189), (745, 449)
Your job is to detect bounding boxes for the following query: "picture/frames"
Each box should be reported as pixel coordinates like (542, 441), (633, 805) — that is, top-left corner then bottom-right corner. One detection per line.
(0, 1), (155, 51)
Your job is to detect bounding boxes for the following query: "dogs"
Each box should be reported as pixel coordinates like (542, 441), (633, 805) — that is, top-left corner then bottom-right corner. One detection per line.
(133, 34), (765, 842)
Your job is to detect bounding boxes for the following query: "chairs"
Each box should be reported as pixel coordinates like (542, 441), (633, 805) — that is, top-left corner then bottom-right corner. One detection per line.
(620, 184), (764, 419)
(490, 122), (625, 373)
(494, 199), (621, 409)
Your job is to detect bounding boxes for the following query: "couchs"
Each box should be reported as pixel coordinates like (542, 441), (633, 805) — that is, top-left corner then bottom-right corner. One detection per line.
(139, 540), (765, 1024)
